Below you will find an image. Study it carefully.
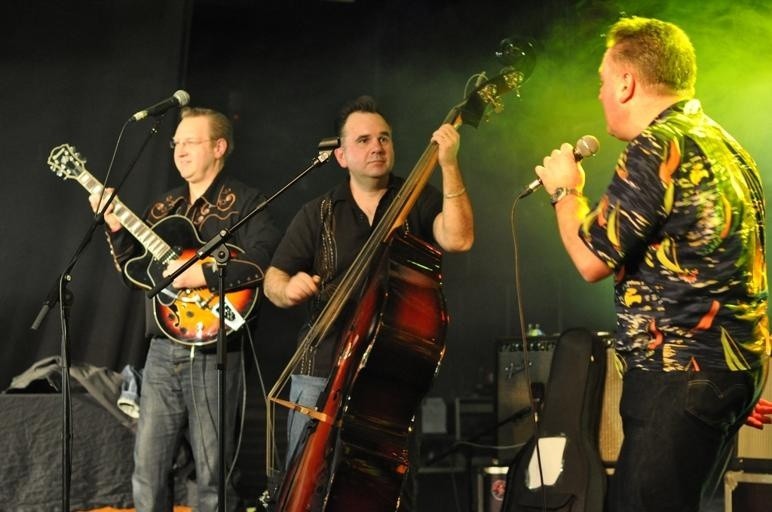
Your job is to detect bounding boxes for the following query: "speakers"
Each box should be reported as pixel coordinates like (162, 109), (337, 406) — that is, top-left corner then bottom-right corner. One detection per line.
(455, 394), (495, 442)
(494, 335), (621, 464)
(401, 462), (474, 511)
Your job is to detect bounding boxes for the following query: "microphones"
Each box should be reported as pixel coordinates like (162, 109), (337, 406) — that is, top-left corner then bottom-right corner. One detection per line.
(529, 382), (545, 427)
(132, 89), (189, 123)
(517, 133), (600, 198)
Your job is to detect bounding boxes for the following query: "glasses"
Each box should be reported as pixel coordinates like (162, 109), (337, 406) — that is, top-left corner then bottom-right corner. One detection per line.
(168, 136), (210, 149)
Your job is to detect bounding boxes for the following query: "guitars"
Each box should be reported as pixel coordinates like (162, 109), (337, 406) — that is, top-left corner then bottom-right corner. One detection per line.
(46, 140), (260, 353)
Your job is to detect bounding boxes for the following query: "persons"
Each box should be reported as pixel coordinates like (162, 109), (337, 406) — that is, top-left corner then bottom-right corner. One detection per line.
(263, 98), (475, 474)
(89, 108), (284, 512)
(534, 17), (772, 512)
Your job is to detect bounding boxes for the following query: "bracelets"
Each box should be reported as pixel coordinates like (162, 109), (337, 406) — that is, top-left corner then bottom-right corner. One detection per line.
(443, 188), (466, 199)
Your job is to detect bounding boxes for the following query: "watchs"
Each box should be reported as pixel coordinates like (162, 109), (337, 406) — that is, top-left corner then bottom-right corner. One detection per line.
(550, 186), (583, 209)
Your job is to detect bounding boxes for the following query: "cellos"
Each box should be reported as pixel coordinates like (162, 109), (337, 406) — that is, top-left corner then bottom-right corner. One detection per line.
(265, 32), (532, 512)
